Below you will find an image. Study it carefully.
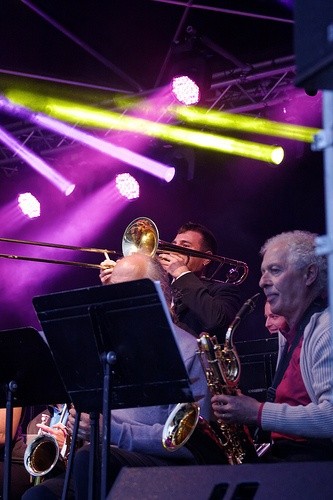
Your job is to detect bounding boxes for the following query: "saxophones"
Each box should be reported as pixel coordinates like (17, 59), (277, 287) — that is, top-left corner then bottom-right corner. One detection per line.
(24, 404), (83, 477)
(161, 293), (270, 466)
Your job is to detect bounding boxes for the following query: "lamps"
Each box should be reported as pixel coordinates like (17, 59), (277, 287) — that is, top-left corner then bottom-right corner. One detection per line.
(171, 36), (215, 105)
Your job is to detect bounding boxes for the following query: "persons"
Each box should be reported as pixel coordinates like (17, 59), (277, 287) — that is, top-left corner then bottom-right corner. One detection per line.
(98, 223), (236, 349)
(12, 403), (88, 463)
(211, 229), (333, 461)
(264, 299), (289, 371)
(23, 254), (210, 500)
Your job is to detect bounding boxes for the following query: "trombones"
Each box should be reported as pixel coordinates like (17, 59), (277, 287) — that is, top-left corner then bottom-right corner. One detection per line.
(0, 217), (249, 285)
